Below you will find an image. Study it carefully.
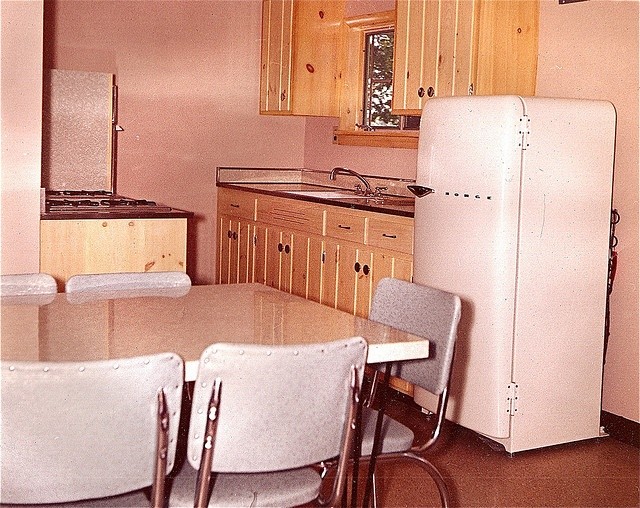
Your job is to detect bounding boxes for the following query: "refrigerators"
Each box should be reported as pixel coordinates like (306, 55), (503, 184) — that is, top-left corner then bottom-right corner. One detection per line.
(411, 91), (621, 461)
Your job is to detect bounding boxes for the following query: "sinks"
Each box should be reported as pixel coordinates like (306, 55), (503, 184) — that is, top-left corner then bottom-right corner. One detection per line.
(284, 191), (379, 199)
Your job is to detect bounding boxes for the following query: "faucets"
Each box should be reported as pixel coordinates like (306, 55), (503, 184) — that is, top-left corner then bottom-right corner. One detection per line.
(330, 167), (374, 197)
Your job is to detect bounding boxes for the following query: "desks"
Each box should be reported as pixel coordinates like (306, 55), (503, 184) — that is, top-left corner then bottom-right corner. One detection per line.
(1, 281), (430, 508)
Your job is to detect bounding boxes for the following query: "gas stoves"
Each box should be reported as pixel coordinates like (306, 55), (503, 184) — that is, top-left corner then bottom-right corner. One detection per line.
(43, 188), (168, 210)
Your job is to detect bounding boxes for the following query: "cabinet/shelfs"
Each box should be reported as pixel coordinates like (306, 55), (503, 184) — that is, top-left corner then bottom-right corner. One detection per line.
(391, 0), (541, 117)
(365, 218), (415, 254)
(216, 218), (257, 284)
(257, 198), (327, 237)
(258, 0), (349, 119)
(217, 192), (258, 221)
(322, 242), (414, 398)
(253, 226), (325, 305)
(326, 212), (369, 245)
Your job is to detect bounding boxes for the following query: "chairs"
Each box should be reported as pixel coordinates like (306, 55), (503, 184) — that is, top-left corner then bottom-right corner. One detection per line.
(1, 274), (58, 296)
(0, 353), (178, 508)
(314, 276), (462, 508)
(65, 271), (191, 293)
(167, 337), (368, 508)
